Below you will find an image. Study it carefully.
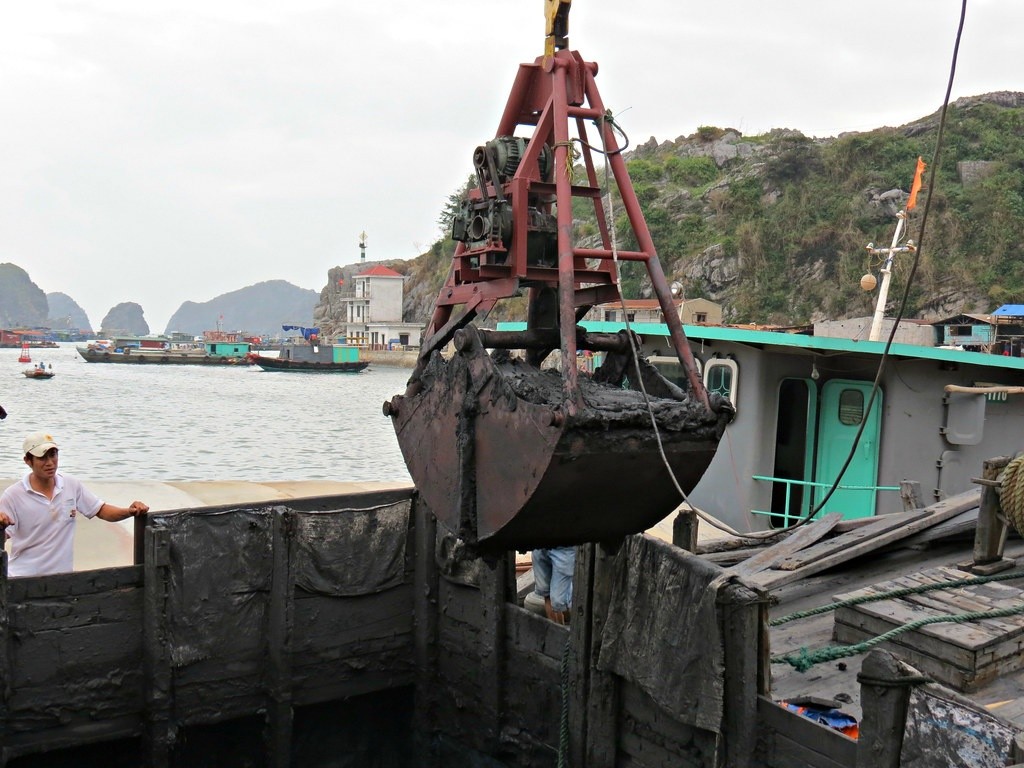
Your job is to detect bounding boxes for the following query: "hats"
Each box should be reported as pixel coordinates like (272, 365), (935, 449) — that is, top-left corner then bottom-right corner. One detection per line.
(21, 431), (59, 458)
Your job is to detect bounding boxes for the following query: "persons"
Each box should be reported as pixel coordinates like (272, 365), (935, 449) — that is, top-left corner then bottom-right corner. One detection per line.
(34, 362), (53, 375)
(0, 431), (150, 578)
(576, 349), (594, 371)
(532, 545), (578, 627)
(1003, 349), (1009, 356)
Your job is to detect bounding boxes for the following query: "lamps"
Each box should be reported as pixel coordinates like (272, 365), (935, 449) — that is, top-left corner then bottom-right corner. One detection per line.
(671, 281), (683, 296)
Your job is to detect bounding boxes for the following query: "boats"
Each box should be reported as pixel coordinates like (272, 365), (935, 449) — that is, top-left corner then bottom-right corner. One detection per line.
(18, 338), (31, 362)
(246, 324), (372, 373)
(74, 344), (225, 364)
(22, 369), (55, 380)
(30, 343), (60, 348)
(203, 319), (251, 356)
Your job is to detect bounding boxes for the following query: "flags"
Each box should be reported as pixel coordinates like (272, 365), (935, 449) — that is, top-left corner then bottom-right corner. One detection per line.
(906, 157), (927, 210)
